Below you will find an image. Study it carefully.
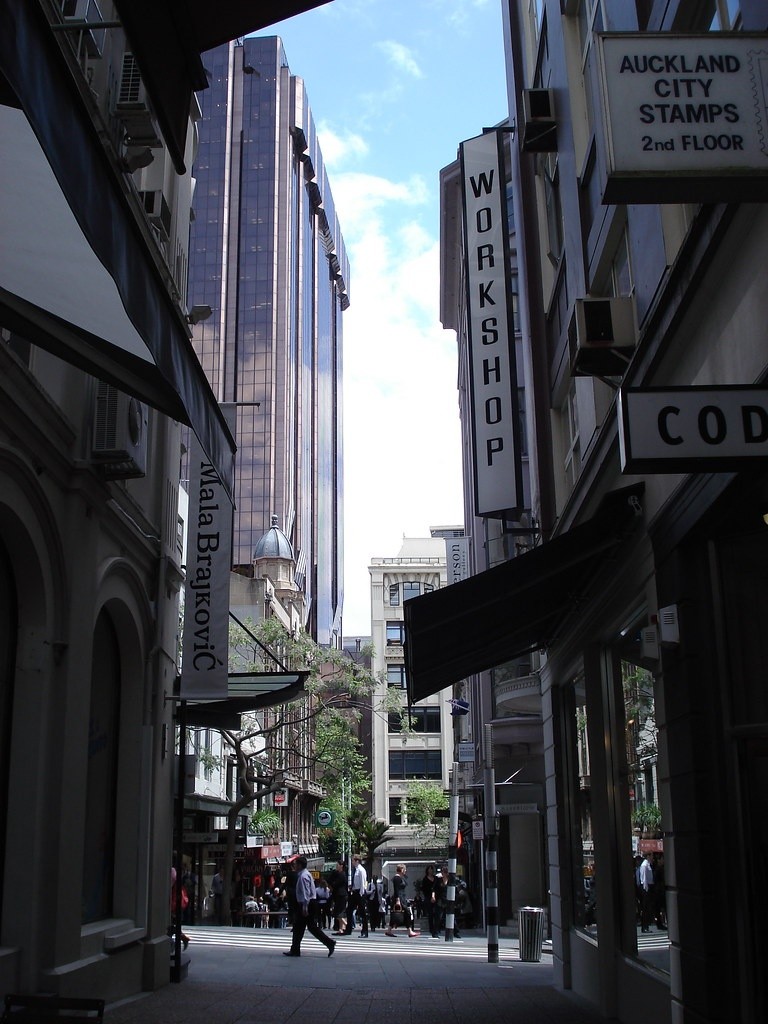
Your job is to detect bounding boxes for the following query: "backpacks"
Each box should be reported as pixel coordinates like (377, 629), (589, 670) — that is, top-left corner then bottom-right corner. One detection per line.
(172, 880), (188, 912)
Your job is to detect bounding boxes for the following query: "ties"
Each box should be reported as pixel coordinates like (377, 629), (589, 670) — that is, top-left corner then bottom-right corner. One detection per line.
(374, 885), (378, 900)
(351, 868), (356, 886)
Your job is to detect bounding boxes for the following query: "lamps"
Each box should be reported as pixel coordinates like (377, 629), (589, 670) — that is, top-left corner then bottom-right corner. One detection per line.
(122, 146), (154, 174)
(185, 304), (212, 325)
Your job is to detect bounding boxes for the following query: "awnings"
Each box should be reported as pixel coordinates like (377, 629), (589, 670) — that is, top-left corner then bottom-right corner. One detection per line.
(403, 507), (626, 708)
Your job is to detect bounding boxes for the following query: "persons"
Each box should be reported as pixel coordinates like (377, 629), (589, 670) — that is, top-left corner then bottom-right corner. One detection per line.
(634, 851), (669, 933)
(211, 854), (466, 957)
(171, 867), (189, 952)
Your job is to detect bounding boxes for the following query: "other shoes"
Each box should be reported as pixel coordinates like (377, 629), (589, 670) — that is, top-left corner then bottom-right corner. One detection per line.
(432, 933), (439, 938)
(339, 930), (351, 935)
(408, 932), (420, 938)
(454, 933), (461, 938)
(385, 932), (397, 937)
(182, 936), (190, 950)
(358, 932), (368, 938)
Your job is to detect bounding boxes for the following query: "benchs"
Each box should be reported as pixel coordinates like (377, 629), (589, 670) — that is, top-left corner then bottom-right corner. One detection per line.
(0, 993), (105, 1024)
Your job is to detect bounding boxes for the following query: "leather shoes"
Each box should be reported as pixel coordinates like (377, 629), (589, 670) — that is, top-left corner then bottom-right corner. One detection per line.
(641, 929), (653, 933)
(657, 925), (668, 930)
(283, 950), (300, 956)
(327, 941), (336, 957)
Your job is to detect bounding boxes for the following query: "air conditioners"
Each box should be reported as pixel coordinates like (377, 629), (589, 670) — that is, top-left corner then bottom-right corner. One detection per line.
(60, 0), (107, 59)
(567, 297), (635, 378)
(92, 376), (147, 477)
(118, 52), (167, 149)
(138, 189), (171, 242)
(519, 88), (557, 153)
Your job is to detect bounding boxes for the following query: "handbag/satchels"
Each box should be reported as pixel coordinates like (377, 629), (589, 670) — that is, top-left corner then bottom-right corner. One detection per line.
(390, 904), (404, 926)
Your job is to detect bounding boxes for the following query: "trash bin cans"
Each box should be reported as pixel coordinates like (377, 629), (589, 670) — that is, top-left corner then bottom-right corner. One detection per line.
(517, 907), (545, 962)
